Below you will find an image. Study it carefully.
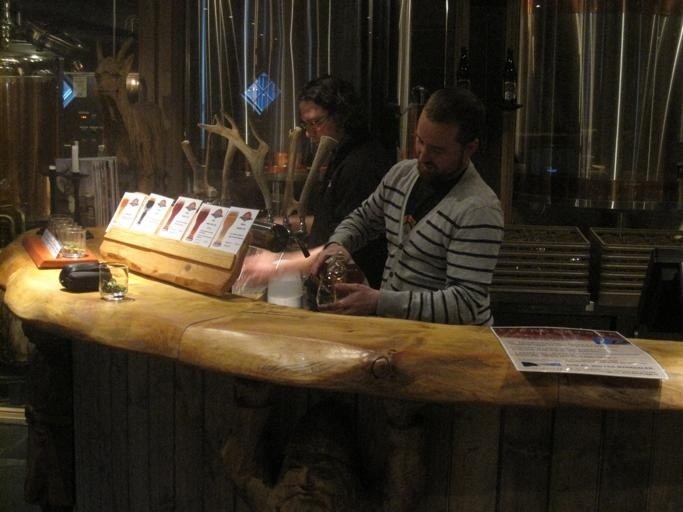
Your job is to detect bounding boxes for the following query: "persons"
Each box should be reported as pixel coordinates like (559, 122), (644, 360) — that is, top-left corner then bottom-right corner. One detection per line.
(306, 82), (508, 329)
(235, 71), (389, 307)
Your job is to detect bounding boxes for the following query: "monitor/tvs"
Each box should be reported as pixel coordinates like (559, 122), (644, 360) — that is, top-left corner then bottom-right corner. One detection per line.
(62, 74), (78, 110)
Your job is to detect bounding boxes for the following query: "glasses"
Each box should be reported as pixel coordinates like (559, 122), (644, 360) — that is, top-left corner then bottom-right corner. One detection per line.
(299, 112), (334, 131)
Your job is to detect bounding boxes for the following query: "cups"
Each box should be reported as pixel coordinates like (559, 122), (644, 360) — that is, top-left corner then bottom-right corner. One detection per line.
(316, 278), (352, 314)
(99, 262), (129, 301)
(48, 214), (87, 259)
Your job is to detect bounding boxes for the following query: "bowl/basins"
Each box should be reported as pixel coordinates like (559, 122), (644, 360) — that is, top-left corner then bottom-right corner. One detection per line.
(21, 17), (83, 62)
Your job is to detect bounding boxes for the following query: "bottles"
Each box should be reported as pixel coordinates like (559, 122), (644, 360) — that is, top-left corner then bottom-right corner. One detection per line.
(302, 255), (350, 310)
